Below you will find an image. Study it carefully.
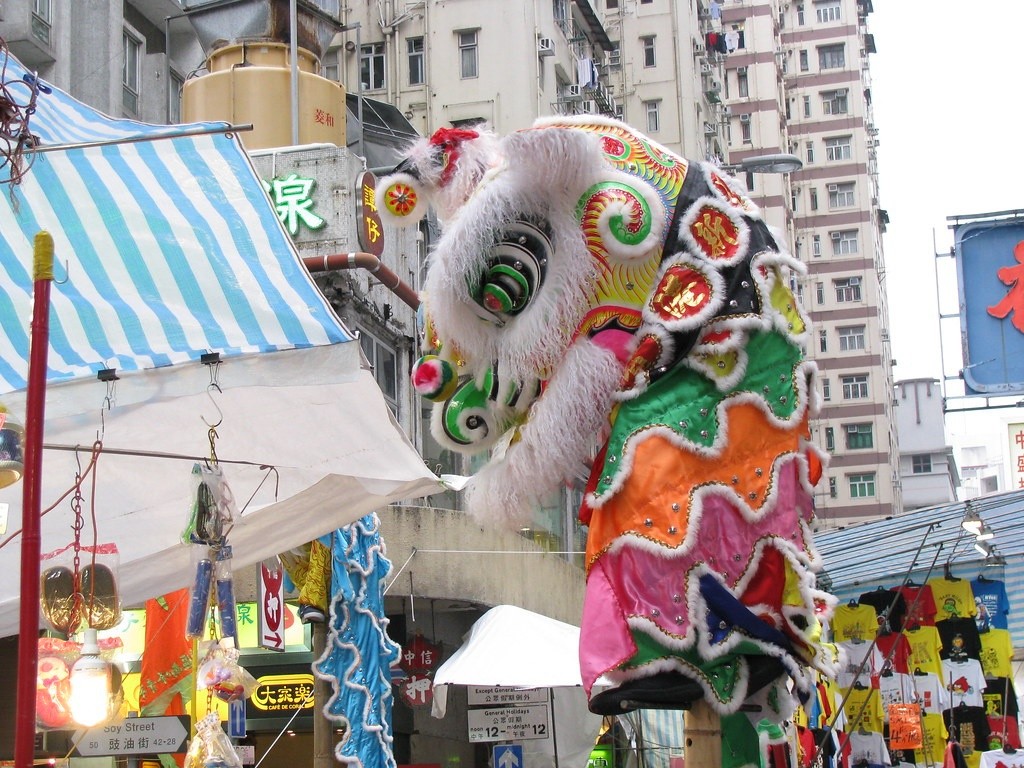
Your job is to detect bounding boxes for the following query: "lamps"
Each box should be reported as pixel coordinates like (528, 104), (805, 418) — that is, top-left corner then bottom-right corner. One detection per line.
(961, 503), (995, 556)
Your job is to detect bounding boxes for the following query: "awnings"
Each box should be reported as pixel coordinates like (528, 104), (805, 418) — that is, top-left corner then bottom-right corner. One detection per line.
(0, 48), (473, 640)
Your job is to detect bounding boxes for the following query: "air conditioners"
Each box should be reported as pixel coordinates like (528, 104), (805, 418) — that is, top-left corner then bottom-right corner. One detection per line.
(565, 84), (584, 102)
(857, 3), (880, 208)
(592, 81), (608, 102)
(582, 100), (599, 115)
(848, 425), (857, 433)
(829, 184), (838, 193)
(694, 8), (732, 137)
(850, 278), (859, 287)
(737, 66), (746, 76)
(600, 94), (616, 117)
(740, 114), (750, 124)
(831, 231), (841, 241)
(538, 38), (556, 57)
(836, 90), (846, 98)
(881, 328), (902, 488)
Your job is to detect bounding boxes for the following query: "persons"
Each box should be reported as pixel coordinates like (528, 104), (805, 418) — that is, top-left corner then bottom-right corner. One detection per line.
(279, 540), (332, 622)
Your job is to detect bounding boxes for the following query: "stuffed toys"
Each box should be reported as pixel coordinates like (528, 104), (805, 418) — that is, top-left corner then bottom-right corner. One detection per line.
(391, 114), (847, 768)
(276, 512), (393, 768)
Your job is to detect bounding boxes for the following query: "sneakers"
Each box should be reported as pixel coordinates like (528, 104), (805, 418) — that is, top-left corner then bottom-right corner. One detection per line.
(589, 670), (703, 714)
(743, 654), (786, 700)
(297, 603), (325, 625)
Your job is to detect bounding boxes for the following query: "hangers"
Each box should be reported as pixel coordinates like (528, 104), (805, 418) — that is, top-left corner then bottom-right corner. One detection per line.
(779, 567), (1024, 768)
